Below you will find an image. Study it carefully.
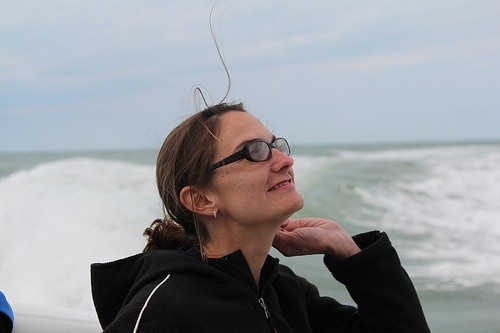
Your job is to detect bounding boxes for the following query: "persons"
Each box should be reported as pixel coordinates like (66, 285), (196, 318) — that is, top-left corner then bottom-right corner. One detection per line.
(90, 7), (430, 333)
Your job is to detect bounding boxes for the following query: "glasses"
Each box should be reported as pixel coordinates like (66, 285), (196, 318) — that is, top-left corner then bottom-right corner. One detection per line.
(206, 137), (291, 172)
